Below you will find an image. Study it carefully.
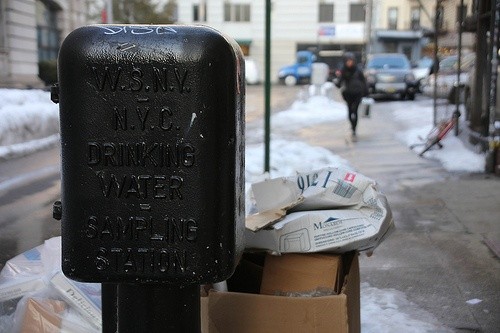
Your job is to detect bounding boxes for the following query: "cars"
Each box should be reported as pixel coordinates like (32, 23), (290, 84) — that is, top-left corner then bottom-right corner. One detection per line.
(420, 51), (475, 104)
(413, 55), (436, 89)
(362, 52), (416, 102)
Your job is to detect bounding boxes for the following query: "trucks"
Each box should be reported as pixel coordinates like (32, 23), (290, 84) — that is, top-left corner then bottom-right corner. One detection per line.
(278, 49), (354, 87)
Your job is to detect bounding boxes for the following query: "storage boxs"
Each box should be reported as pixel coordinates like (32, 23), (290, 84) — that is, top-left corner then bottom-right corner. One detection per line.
(198, 161), (395, 332)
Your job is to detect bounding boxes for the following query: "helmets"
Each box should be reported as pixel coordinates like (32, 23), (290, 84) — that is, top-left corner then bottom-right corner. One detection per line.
(344, 52), (356, 60)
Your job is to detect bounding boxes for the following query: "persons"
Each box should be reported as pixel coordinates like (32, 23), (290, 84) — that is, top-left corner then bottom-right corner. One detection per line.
(334, 51), (369, 143)
